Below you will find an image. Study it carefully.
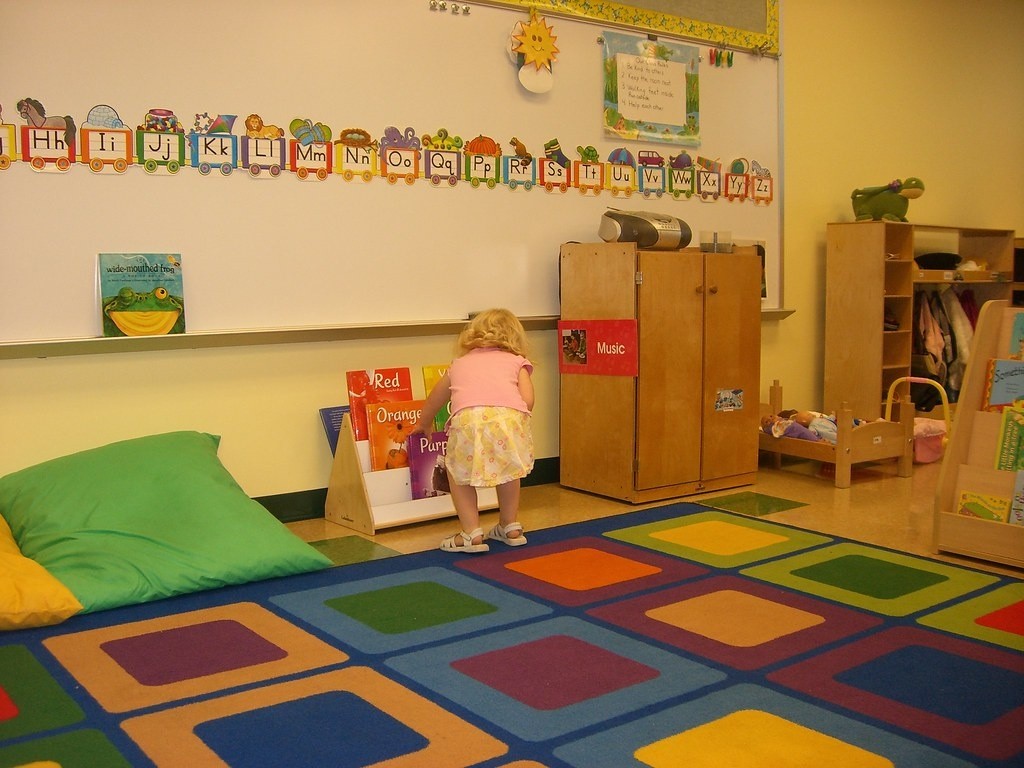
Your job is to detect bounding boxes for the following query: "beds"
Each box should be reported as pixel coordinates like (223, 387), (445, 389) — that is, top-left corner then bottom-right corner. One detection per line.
(761, 379), (915, 488)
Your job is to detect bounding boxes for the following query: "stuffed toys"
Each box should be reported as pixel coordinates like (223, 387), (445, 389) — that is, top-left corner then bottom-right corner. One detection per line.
(851, 177), (925, 223)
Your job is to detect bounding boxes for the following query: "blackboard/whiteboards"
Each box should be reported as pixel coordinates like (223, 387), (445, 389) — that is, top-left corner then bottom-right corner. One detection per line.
(1, 1), (783, 348)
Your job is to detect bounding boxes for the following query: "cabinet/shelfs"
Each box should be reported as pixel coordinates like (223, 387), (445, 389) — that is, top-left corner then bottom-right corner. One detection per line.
(933, 299), (1024, 569)
(559, 242), (762, 505)
(325, 413), (501, 535)
(821, 221), (1024, 466)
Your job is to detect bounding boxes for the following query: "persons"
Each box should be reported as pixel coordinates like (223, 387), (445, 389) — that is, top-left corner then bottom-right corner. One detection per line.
(408, 309), (535, 552)
(761, 408), (861, 446)
(564, 332), (586, 357)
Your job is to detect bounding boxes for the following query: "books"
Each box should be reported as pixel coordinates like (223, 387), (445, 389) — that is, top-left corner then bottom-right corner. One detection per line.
(346, 362), (452, 501)
(956, 312), (1024, 528)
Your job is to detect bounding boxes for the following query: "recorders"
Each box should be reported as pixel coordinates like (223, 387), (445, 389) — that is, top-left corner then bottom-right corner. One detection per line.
(598, 207), (692, 251)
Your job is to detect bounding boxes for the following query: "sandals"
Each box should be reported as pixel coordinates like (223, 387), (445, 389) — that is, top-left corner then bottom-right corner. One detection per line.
(440, 528), (489, 552)
(486, 522), (527, 546)
